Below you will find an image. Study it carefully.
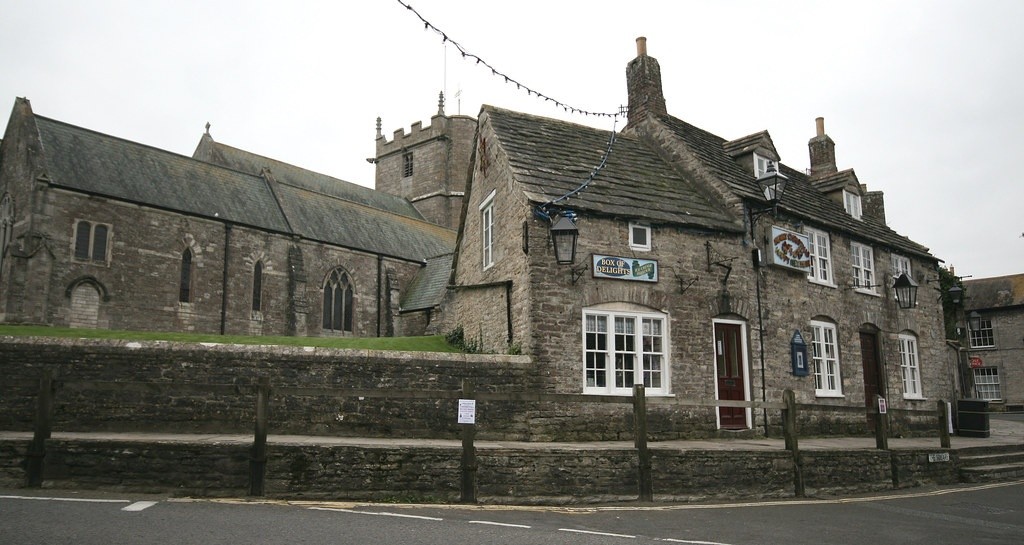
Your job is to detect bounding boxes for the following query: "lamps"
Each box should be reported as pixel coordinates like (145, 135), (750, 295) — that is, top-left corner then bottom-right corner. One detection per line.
(935, 283), (962, 304)
(550, 210), (580, 265)
(750, 160), (789, 225)
(967, 311), (982, 331)
(893, 273), (919, 309)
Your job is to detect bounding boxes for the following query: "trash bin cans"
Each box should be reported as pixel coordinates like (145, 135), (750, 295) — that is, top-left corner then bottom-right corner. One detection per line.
(957, 399), (990, 438)
